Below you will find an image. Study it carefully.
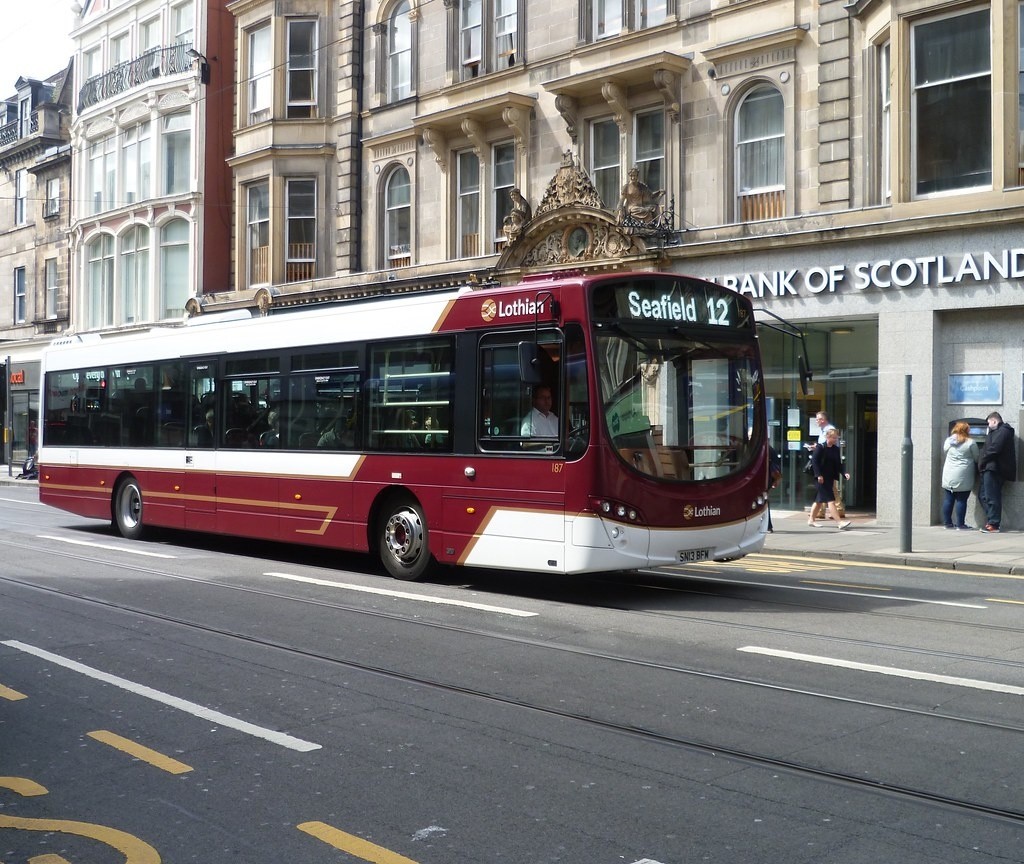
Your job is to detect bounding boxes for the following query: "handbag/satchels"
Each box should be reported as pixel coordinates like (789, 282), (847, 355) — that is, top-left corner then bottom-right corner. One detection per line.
(770, 463), (782, 480)
(803, 444), (825, 477)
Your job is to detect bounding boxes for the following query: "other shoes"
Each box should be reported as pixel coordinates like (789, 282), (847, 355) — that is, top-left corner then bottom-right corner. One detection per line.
(808, 521), (823, 527)
(808, 513), (825, 520)
(829, 515), (845, 519)
(768, 529), (773, 533)
(838, 521), (851, 530)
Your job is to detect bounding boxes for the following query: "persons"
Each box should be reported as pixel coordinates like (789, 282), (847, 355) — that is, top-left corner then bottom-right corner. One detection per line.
(748, 427), (781, 533)
(318, 417), (354, 448)
(808, 411), (845, 519)
(807, 424), (851, 529)
(978, 412), (1016, 533)
(519, 385), (586, 461)
(199, 409), (215, 448)
(25, 421), (38, 457)
(941, 421), (980, 531)
(618, 166), (666, 222)
(504, 188), (532, 244)
(135, 378), (146, 392)
(383, 410), (442, 448)
(265, 409), (280, 449)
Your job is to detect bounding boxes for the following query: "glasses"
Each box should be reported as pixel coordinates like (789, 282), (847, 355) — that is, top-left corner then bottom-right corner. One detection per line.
(535, 396), (552, 401)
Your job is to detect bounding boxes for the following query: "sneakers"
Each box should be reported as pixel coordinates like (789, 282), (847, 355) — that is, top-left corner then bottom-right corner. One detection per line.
(944, 524), (956, 530)
(980, 523), (1000, 533)
(956, 525), (973, 531)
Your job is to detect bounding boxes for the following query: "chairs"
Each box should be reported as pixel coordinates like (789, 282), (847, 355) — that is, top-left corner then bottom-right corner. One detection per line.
(132, 391), (527, 454)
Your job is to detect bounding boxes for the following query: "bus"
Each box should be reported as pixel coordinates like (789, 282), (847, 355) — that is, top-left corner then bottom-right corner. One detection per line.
(37, 270), (813, 582)
